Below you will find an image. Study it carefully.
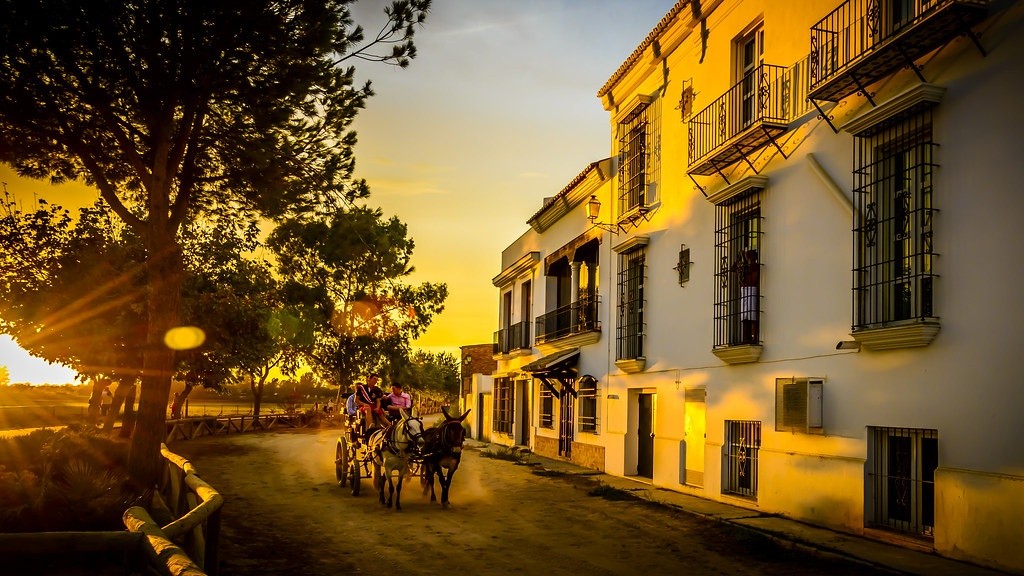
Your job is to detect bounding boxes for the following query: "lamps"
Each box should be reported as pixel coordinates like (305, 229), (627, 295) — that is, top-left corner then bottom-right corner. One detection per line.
(585, 195), (620, 236)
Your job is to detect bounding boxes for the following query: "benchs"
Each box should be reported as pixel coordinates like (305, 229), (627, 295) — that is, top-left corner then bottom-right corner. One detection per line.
(340, 393), (413, 446)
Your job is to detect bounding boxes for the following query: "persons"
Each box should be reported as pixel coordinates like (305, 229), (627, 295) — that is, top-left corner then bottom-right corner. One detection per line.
(327, 398), (334, 418)
(312, 401), (318, 412)
(345, 382), (364, 423)
(382, 382), (412, 418)
(354, 373), (398, 434)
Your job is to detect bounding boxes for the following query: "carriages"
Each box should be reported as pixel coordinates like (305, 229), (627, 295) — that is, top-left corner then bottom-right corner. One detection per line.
(333, 393), (471, 513)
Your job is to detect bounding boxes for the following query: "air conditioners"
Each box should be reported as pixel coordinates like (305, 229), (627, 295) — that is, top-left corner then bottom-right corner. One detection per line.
(782, 383), (823, 428)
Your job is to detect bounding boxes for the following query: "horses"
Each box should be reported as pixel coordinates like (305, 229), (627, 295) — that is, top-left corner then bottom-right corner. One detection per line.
(368, 406), (425, 513)
(420, 406), (471, 510)
